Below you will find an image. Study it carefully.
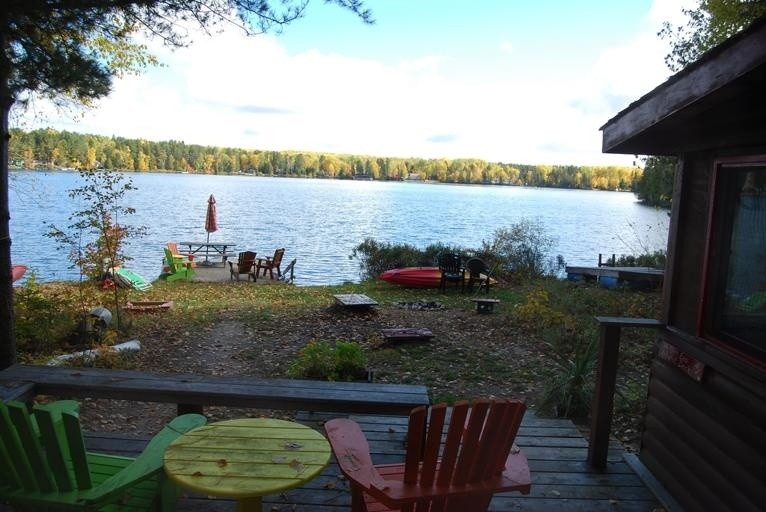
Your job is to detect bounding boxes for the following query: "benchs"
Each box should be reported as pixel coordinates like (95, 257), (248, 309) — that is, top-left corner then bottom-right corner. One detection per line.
(180, 241), (237, 268)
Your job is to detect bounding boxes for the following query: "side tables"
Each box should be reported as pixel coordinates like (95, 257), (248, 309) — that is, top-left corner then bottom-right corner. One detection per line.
(164, 418), (332, 512)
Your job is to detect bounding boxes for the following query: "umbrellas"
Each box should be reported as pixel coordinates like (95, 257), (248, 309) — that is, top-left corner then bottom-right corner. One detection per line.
(203, 193), (218, 262)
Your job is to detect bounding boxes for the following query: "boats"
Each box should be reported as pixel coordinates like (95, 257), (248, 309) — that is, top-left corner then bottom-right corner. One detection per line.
(380, 266), (497, 291)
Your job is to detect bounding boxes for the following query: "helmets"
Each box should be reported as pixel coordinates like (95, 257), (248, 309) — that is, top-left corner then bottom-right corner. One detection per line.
(90, 307), (113, 328)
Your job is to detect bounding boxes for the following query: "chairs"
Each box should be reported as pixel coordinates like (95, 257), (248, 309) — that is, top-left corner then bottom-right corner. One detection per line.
(469, 259), (490, 293)
(1, 399), (207, 511)
(162, 242), (198, 271)
(228, 251), (257, 283)
(257, 248), (284, 282)
(437, 253), (466, 294)
(325, 398), (532, 512)
(159, 249), (193, 282)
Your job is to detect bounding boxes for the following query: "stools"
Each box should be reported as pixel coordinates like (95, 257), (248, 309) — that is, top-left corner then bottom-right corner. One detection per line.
(471, 299), (500, 315)
